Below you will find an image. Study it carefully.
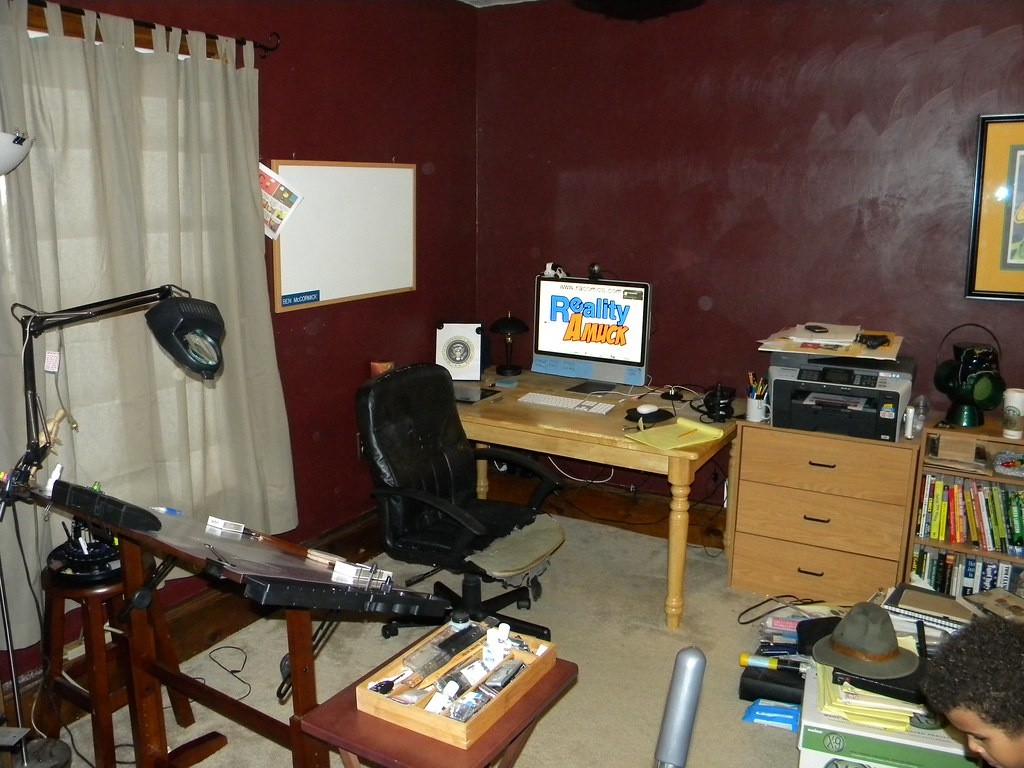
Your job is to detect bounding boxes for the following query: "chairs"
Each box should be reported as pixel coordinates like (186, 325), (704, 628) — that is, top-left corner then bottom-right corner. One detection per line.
(353, 362), (554, 641)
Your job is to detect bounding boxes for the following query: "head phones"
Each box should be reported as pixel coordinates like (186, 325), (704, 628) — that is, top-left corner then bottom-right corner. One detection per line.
(689, 391), (734, 422)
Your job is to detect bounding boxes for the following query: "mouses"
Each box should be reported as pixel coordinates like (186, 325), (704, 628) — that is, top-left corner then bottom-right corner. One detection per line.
(637, 404), (659, 415)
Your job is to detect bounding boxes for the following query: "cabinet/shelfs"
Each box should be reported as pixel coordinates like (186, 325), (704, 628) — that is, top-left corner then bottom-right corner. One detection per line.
(723, 414), (945, 611)
(904, 411), (1024, 606)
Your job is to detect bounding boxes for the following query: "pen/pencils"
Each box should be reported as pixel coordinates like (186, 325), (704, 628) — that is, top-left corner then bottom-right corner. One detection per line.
(747, 371), (768, 400)
(677, 428), (698, 438)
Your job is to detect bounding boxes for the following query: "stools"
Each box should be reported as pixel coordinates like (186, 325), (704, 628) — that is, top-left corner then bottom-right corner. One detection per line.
(39, 565), (197, 768)
(299, 617), (580, 768)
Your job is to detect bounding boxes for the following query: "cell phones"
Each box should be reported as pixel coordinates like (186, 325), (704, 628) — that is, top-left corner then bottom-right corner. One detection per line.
(805, 325), (829, 332)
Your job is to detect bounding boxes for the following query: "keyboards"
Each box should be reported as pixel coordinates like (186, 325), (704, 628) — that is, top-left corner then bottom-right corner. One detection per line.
(517, 391), (616, 415)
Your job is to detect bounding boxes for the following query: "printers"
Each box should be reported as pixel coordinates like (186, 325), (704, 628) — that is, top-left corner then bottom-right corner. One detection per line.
(767, 351), (917, 442)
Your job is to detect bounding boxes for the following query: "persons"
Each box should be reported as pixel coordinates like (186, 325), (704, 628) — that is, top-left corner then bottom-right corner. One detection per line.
(921, 616), (1024, 768)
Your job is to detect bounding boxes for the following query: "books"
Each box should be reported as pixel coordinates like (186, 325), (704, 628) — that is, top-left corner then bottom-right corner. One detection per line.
(767, 473), (1024, 733)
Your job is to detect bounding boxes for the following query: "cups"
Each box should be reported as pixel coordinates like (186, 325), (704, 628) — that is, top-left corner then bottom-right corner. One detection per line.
(1002, 388), (1024, 439)
(746, 397), (771, 422)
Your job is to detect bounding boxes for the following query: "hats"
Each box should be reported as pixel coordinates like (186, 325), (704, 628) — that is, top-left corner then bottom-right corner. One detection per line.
(812, 602), (919, 681)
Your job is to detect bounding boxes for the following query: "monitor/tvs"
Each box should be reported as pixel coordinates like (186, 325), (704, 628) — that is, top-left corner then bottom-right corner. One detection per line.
(531, 276), (654, 396)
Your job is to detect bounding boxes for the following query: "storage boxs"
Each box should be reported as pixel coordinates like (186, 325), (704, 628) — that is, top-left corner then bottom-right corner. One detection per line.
(334, 562), (392, 580)
(205, 516), (246, 532)
(355, 619), (555, 750)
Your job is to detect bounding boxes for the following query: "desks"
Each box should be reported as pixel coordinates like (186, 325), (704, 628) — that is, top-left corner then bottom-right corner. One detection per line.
(441, 362), (744, 631)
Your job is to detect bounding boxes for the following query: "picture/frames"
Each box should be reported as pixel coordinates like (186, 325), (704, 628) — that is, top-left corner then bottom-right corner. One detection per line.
(965, 113), (1024, 304)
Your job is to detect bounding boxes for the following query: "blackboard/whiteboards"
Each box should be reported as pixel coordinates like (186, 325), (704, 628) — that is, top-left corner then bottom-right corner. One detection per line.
(270, 159), (416, 313)
